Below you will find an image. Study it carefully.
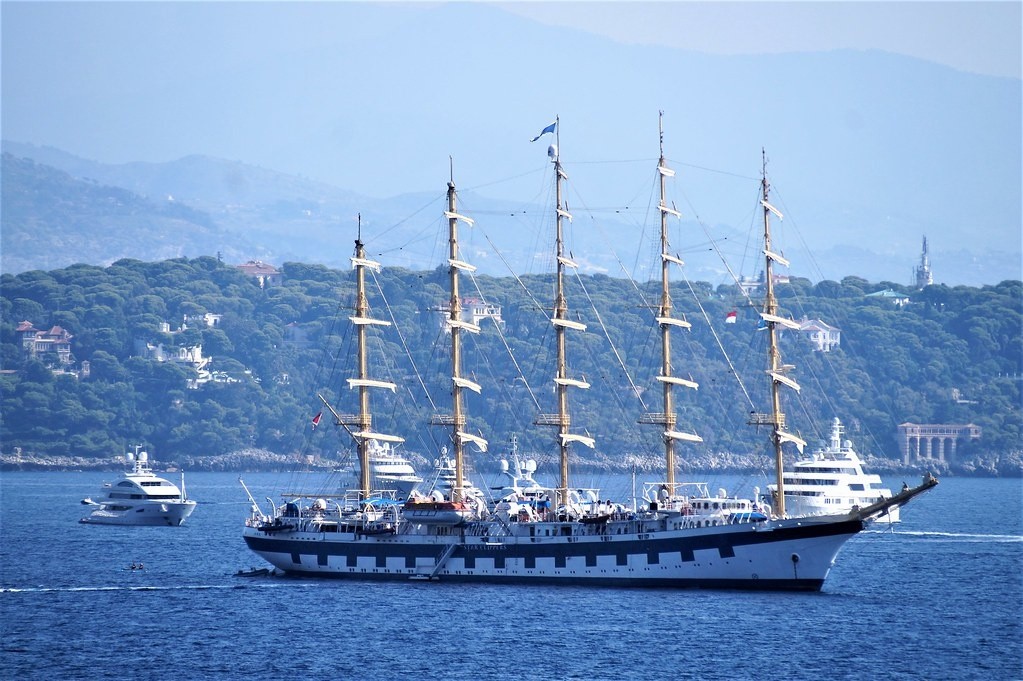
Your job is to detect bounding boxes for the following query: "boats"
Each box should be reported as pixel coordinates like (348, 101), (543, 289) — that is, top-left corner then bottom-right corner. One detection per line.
(395, 446), (482, 526)
(334, 431), (423, 503)
(481, 432), (553, 511)
(78, 441), (197, 528)
(756, 413), (902, 527)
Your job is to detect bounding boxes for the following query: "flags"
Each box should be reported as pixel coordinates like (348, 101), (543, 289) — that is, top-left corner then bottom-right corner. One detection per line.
(725, 311), (736, 324)
(312, 412), (322, 426)
(530, 122), (555, 142)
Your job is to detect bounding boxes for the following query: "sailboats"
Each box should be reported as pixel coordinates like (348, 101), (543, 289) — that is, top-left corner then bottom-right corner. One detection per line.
(233, 110), (940, 594)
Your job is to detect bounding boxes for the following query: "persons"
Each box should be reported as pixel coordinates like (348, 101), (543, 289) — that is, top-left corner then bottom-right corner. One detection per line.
(131, 563), (137, 570)
(139, 563), (143, 570)
